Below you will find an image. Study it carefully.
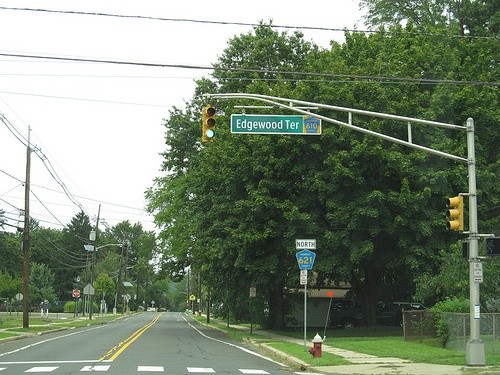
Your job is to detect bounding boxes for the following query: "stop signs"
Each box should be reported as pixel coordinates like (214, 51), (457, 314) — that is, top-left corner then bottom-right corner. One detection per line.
(72, 290), (80, 297)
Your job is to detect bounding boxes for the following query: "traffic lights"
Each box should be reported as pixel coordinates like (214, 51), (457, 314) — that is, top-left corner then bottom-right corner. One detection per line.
(444, 196), (464, 232)
(202, 104), (216, 143)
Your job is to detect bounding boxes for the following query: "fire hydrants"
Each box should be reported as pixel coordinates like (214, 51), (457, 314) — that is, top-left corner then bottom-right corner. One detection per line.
(309, 333), (323, 358)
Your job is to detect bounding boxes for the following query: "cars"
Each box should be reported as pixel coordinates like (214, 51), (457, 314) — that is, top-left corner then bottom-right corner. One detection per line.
(328, 298), (429, 330)
